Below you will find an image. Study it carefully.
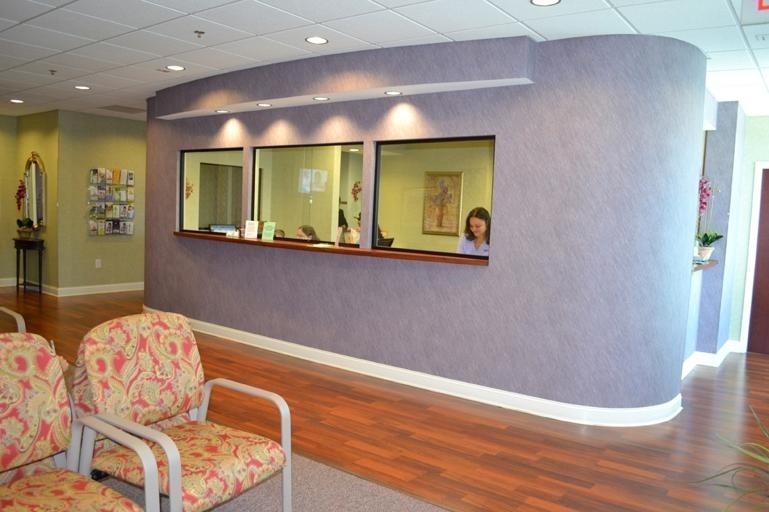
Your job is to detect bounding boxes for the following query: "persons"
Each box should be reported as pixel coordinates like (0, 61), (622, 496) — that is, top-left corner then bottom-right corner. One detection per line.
(274, 228), (285, 238)
(295, 224), (320, 241)
(338, 195), (348, 229)
(454, 206), (492, 258)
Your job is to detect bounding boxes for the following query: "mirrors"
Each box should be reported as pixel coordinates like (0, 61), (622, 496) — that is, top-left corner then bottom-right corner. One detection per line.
(22, 151), (46, 227)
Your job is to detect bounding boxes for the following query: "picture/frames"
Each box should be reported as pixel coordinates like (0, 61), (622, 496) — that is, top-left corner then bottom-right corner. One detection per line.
(421, 170), (464, 237)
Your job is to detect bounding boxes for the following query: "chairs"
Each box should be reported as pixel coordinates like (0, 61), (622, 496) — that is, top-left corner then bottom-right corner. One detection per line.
(0, 332), (161, 512)
(85, 312), (292, 512)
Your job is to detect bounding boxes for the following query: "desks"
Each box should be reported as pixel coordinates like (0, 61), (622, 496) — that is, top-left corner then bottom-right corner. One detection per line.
(12, 237), (44, 293)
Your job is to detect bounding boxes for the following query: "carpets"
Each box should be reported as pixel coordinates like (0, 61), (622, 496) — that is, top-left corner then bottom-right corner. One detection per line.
(95, 452), (452, 512)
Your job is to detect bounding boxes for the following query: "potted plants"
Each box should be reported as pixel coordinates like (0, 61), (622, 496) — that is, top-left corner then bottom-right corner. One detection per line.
(695, 232), (723, 261)
